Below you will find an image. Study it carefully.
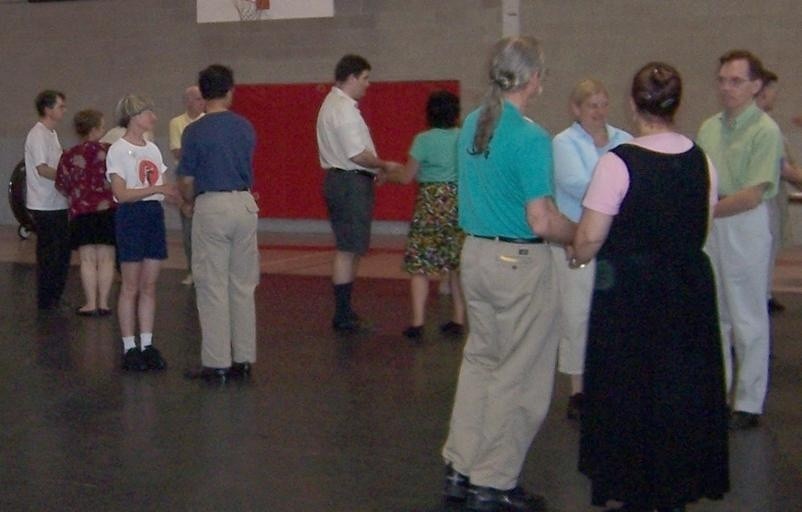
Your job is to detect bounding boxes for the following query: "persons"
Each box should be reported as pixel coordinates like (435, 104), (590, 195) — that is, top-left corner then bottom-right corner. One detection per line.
(315, 52), (403, 337)
(105, 91), (184, 374)
(698, 50), (783, 431)
(547, 78), (634, 414)
(55, 108), (119, 318)
(566, 61), (730, 512)
(179, 63), (260, 387)
(24, 90), (72, 319)
(373, 89), (466, 341)
(756, 70), (802, 315)
(170, 84), (209, 287)
(100, 120), (151, 283)
(440, 38), (579, 512)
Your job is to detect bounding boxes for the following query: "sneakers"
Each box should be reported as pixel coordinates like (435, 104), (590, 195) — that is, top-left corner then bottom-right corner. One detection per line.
(139, 344), (166, 369)
(120, 347), (149, 370)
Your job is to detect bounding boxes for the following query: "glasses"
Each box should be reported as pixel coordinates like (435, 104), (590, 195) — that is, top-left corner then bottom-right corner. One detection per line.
(713, 74), (751, 86)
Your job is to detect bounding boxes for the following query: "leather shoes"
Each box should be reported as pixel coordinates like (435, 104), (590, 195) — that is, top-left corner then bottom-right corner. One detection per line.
(97, 307), (113, 316)
(439, 319), (467, 335)
(184, 366), (232, 385)
(401, 324), (426, 337)
(567, 392), (585, 420)
(728, 410), (760, 431)
(75, 304), (98, 317)
(442, 466), (471, 500)
(232, 361), (252, 378)
(466, 483), (547, 511)
(768, 296), (787, 312)
(335, 315), (374, 334)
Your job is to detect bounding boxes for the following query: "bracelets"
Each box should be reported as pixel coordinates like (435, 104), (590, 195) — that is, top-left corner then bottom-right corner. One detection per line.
(571, 255), (591, 269)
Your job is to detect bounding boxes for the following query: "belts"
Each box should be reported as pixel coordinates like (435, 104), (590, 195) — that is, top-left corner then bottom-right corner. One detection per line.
(334, 165), (374, 179)
(466, 233), (545, 244)
(194, 187), (248, 198)
